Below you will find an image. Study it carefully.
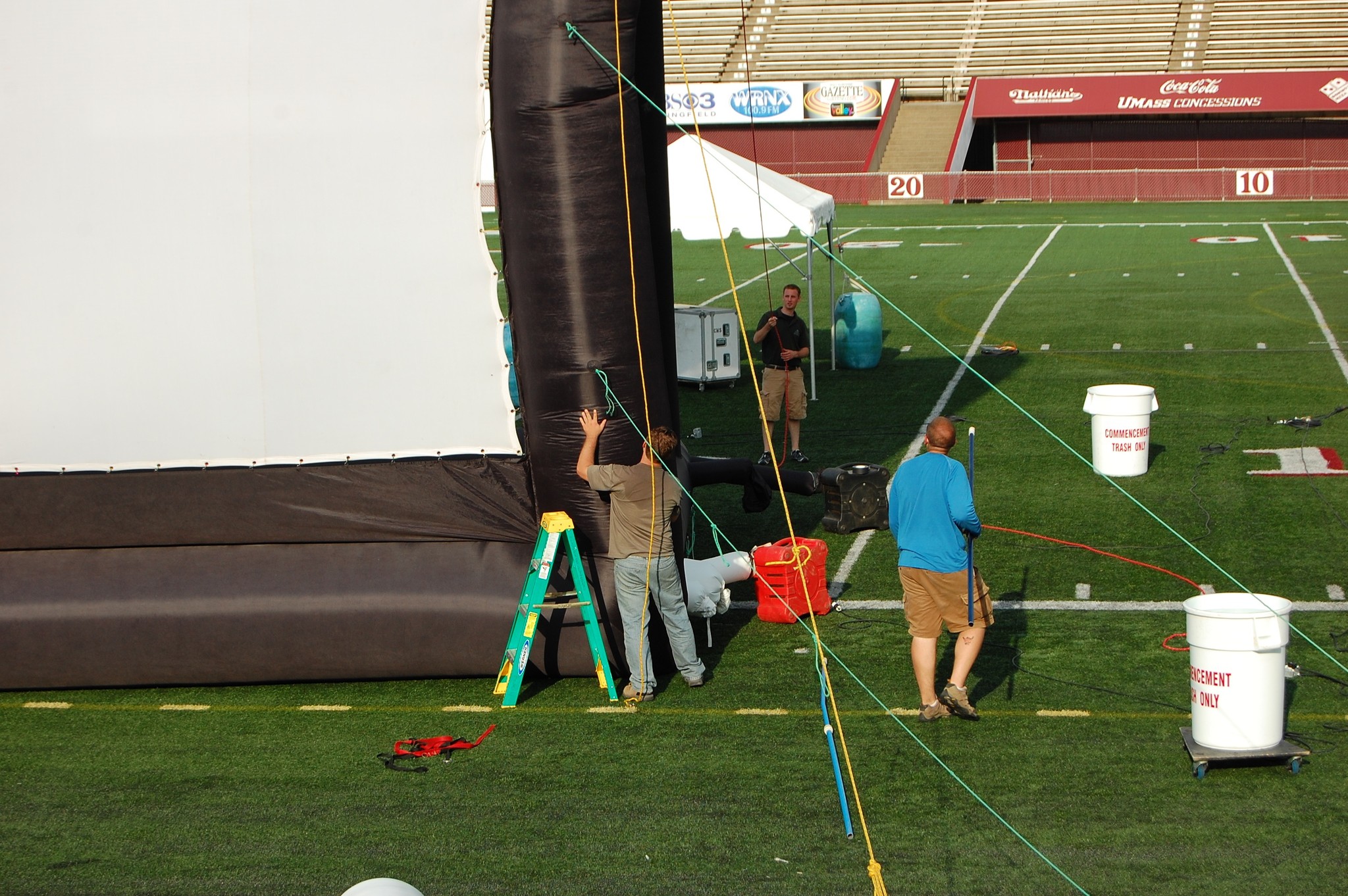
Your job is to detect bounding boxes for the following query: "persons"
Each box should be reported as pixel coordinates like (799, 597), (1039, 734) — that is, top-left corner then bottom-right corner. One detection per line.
(888, 416), (995, 722)
(576, 409), (706, 701)
(753, 284), (810, 465)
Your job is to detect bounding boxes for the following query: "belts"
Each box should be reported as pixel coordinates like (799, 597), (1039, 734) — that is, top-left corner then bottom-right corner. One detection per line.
(765, 365), (798, 370)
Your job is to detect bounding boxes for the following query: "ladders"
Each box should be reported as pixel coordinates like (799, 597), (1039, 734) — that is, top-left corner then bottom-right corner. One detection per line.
(492, 512), (618, 709)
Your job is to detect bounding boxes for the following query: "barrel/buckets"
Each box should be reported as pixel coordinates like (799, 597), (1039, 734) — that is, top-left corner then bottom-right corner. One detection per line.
(1083, 384), (1159, 477)
(1182, 592), (1293, 751)
(834, 292), (883, 371)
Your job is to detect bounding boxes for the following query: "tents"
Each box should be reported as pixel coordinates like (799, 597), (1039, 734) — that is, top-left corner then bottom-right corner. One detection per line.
(666, 134), (836, 401)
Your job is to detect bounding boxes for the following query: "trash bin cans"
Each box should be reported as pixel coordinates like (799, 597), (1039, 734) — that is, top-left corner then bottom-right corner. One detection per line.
(1182, 591), (1294, 749)
(1082, 384), (1159, 477)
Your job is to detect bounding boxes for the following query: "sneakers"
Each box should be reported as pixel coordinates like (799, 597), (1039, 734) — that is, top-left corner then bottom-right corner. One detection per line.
(758, 451), (772, 465)
(918, 694), (953, 722)
(937, 678), (980, 722)
(790, 448), (809, 463)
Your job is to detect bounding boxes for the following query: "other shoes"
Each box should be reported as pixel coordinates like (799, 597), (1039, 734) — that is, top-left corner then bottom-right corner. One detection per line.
(684, 673), (705, 687)
(623, 682), (654, 702)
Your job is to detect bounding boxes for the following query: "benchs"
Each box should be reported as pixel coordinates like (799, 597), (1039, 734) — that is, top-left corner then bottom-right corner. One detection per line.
(662, 0), (1348, 98)
